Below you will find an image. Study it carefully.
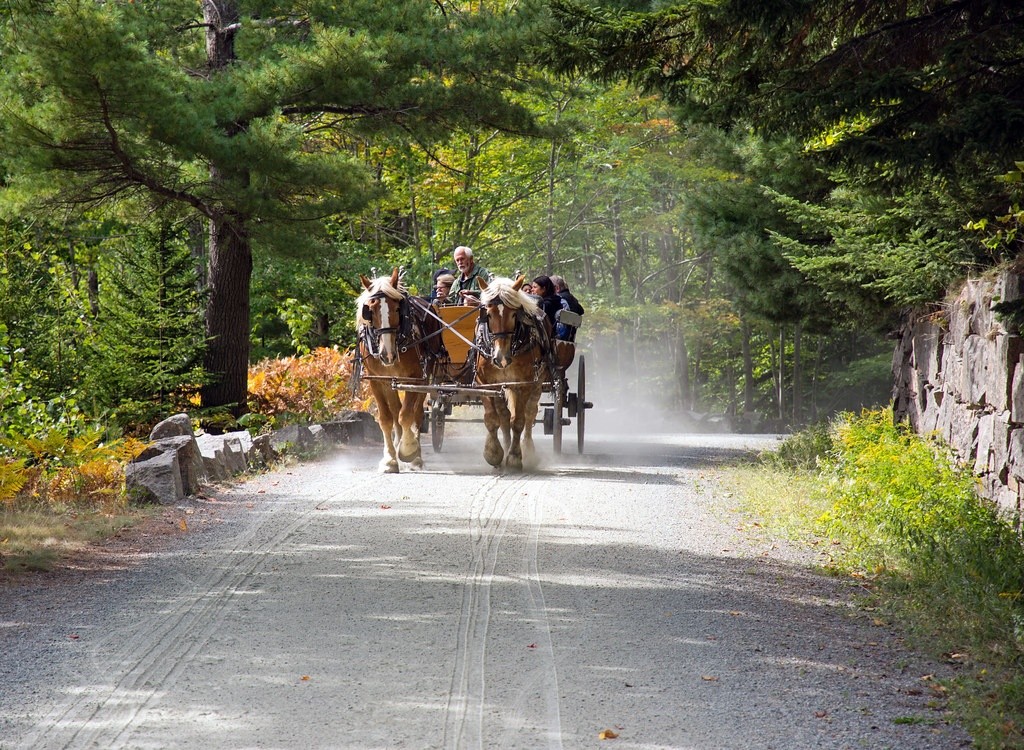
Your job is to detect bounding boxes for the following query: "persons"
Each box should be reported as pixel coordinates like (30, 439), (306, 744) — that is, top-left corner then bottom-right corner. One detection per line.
(429, 269), (457, 303)
(436, 246), (494, 307)
(521, 284), (532, 294)
(530, 275), (584, 343)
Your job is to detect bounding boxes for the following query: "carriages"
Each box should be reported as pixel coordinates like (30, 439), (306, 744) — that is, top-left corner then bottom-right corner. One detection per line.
(352, 268), (593, 474)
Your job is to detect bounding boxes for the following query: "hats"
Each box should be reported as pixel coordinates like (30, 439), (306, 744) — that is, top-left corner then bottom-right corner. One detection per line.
(432, 268), (458, 285)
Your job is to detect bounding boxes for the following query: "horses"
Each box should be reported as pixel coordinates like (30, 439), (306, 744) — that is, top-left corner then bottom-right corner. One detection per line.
(476, 275), (550, 475)
(355, 265), (440, 474)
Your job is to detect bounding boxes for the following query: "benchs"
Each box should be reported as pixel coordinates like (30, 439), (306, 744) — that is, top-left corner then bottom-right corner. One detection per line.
(544, 309), (582, 383)
